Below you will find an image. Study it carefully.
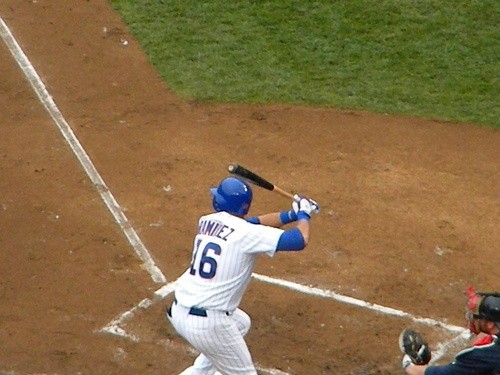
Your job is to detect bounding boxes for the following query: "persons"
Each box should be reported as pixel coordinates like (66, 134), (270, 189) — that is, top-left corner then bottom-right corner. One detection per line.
(166, 174), (319, 375)
(399, 287), (500, 374)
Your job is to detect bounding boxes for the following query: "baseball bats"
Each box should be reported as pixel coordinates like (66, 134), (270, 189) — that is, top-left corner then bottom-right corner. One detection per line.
(227, 163), (301, 200)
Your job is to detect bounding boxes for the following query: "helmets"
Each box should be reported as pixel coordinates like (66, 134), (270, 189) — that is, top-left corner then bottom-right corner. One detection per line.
(466, 293), (500, 335)
(209, 177), (252, 216)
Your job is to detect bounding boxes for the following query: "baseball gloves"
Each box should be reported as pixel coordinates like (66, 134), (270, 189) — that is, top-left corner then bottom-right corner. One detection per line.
(398, 325), (432, 366)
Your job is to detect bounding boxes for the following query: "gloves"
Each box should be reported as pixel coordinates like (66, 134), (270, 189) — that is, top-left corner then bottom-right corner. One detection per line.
(292, 194), (311, 218)
(308, 198), (320, 215)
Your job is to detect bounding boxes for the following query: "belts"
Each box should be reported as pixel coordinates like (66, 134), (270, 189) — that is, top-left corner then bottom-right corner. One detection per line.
(174, 298), (229, 316)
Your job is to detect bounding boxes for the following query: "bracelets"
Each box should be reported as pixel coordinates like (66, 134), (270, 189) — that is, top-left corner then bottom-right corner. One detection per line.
(279, 211), (297, 223)
